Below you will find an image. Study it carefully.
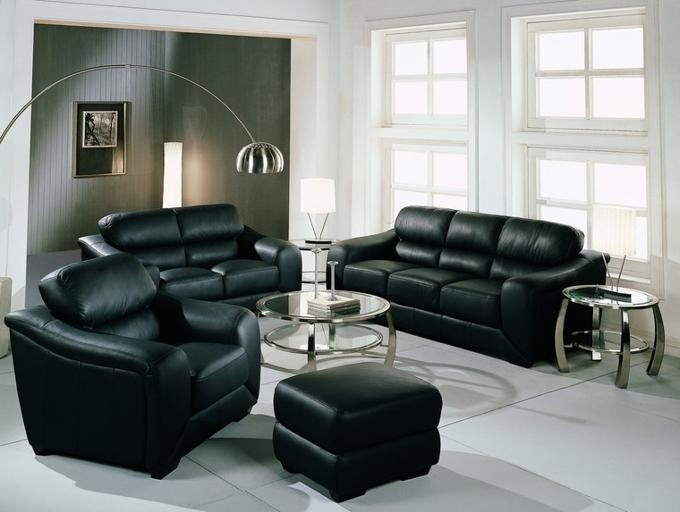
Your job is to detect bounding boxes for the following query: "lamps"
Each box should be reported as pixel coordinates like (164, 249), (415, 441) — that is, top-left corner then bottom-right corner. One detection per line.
(0, 64), (285, 175)
(300, 178), (335, 243)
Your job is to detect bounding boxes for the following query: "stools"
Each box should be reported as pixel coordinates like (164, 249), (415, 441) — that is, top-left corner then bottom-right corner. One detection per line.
(270, 360), (444, 503)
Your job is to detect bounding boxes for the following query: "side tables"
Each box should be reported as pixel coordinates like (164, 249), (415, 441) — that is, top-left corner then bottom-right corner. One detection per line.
(554, 285), (665, 389)
(289, 238), (336, 289)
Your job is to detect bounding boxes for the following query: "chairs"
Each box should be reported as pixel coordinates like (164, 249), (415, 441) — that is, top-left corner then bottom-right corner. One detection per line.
(2, 252), (262, 480)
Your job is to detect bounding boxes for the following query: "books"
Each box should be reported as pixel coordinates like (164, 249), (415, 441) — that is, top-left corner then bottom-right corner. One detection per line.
(309, 293), (360, 316)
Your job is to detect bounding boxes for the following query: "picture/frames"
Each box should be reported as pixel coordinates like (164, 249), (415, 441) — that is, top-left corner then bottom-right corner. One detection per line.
(71, 100), (127, 179)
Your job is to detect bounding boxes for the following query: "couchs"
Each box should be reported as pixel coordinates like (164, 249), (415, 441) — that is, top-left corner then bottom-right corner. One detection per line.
(326, 204), (612, 370)
(78, 203), (302, 317)
